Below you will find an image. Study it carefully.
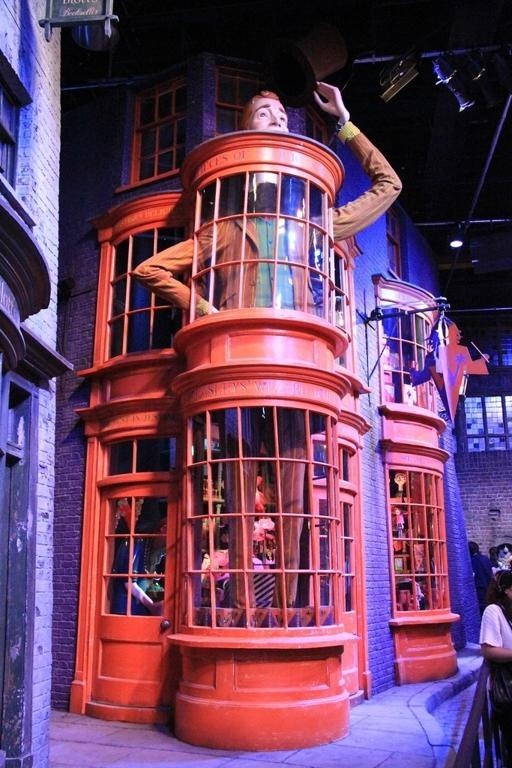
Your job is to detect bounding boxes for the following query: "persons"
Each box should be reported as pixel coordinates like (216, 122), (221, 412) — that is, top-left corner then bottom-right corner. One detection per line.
(468, 541), (496, 618)
(131, 81), (404, 609)
(110, 511), (163, 615)
(478, 569), (512, 768)
(489, 547), (499, 567)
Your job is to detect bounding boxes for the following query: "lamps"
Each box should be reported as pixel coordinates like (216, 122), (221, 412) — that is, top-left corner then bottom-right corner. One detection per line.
(447, 222), (468, 249)
(431, 52), (486, 113)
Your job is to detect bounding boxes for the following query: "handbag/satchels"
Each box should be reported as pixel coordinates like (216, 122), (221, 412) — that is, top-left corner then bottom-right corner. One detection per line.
(490, 660), (512, 713)
